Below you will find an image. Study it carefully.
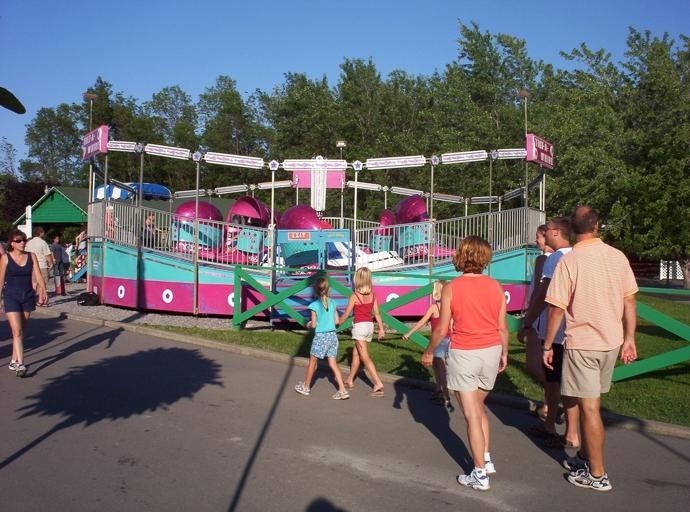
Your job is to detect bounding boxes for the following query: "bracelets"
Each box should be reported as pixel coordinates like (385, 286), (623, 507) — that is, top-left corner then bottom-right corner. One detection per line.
(542, 346), (552, 351)
(521, 323), (532, 330)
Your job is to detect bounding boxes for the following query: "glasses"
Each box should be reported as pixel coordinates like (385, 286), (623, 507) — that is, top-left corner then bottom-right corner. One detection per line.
(12, 239), (28, 243)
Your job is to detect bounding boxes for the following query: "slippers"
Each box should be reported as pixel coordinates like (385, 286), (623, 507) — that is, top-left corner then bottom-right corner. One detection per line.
(343, 380), (354, 390)
(368, 389), (385, 398)
(528, 422), (554, 437)
(543, 435), (577, 448)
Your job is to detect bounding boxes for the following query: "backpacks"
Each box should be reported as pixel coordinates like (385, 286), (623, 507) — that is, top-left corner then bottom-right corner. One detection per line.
(76, 291), (99, 307)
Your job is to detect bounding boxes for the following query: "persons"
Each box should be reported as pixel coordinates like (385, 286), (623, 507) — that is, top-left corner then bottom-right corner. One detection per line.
(143, 211), (162, 247)
(542, 206), (640, 491)
(420, 235), (508, 490)
(338, 266), (385, 397)
(522, 224), (559, 423)
(295, 277), (350, 400)
(517, 215), (580, 450)
(226, 216), (241, 254)
(402, 280), (455, 414)
(2, 224), (89, 372)
(105, 206), (117, 239)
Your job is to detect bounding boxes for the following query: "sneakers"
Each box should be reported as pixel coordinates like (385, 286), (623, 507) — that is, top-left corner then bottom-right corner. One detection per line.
(8, 362), (19, 371)
(472, 461), (497, 475)
(563, 451), (590, 473)
(15, 366), (27, 377)
(457, 472), (491, 491)
(568, 469), (613, 492)
(294, 383), (311, 396)
(331, 389), (351, 400)
